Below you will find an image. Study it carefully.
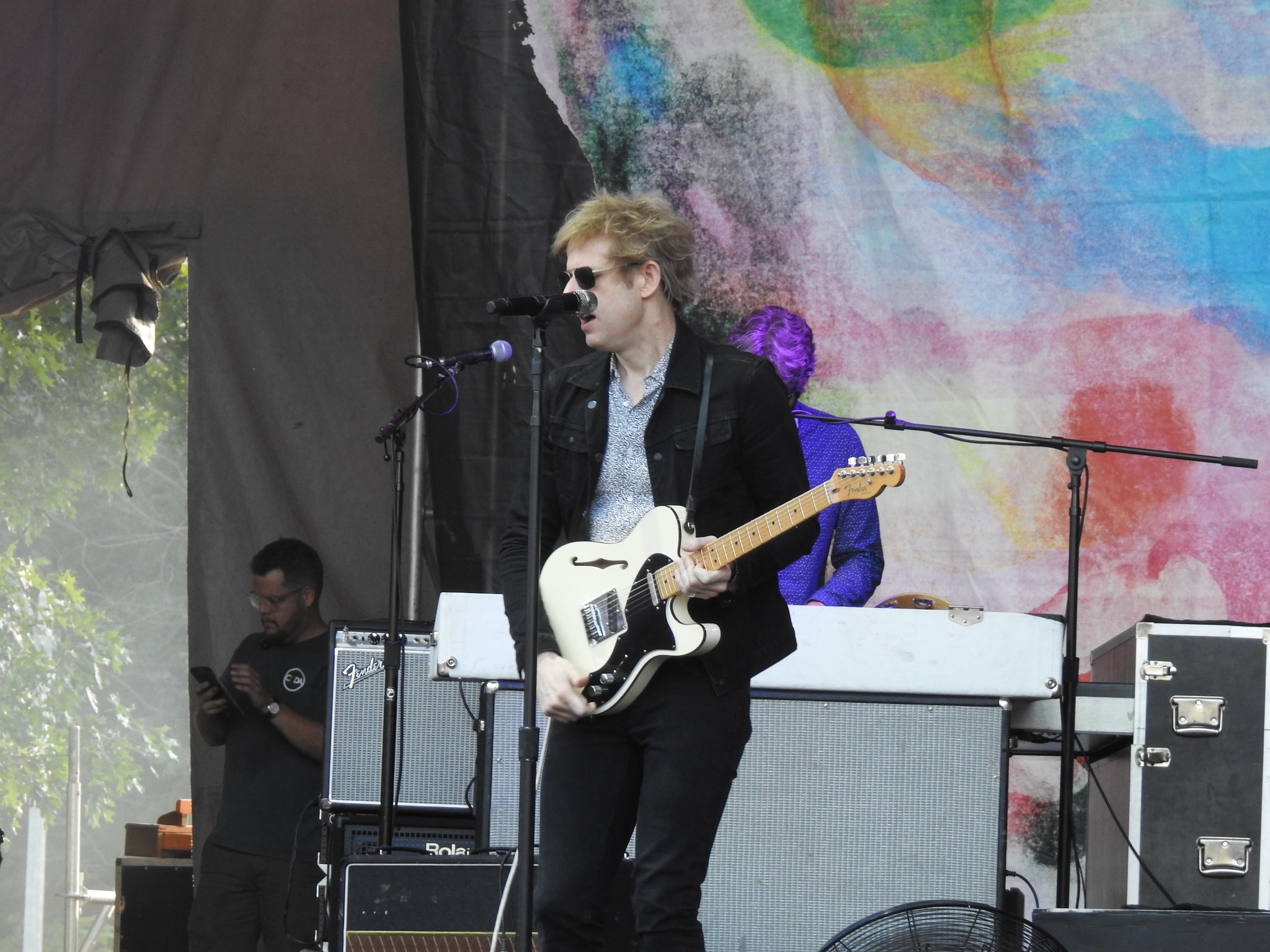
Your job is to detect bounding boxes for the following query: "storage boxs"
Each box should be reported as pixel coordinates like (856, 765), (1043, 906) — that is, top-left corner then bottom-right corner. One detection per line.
(1090, 622), (1270, 913)
(113, 856), (194, 952)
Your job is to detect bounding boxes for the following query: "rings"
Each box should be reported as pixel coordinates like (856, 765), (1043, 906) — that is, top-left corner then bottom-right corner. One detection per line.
(687, 593), (695, 597)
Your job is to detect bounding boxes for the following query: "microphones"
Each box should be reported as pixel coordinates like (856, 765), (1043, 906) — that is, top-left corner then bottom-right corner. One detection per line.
(422, 339), (513, 367)
(485, 290), (599, 317)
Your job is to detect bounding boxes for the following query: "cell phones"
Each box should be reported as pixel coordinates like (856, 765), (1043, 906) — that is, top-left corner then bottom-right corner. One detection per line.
(191, 666), (248, 716)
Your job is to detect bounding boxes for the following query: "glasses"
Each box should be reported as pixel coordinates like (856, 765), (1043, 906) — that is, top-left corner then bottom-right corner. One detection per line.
(558, 263), (637, 288)
(249, 587), (304, 611)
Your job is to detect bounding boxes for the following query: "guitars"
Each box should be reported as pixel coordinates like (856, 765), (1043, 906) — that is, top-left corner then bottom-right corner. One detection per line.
(538, 454), (905, 714)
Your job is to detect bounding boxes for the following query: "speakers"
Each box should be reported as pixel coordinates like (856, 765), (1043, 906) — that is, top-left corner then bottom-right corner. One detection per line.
(324, 621), (506, 809)
(476, 684), (1006, 952)
(339, 850), (542, 952)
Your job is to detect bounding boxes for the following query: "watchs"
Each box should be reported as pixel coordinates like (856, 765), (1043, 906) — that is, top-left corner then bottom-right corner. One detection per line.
(261, 695), (280, 719)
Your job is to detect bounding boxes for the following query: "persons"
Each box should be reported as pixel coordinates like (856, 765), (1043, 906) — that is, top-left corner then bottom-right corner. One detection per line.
(188, 537), (330, 952)
(499, 194), (819, 952)
(725, 307), (885, 607)
